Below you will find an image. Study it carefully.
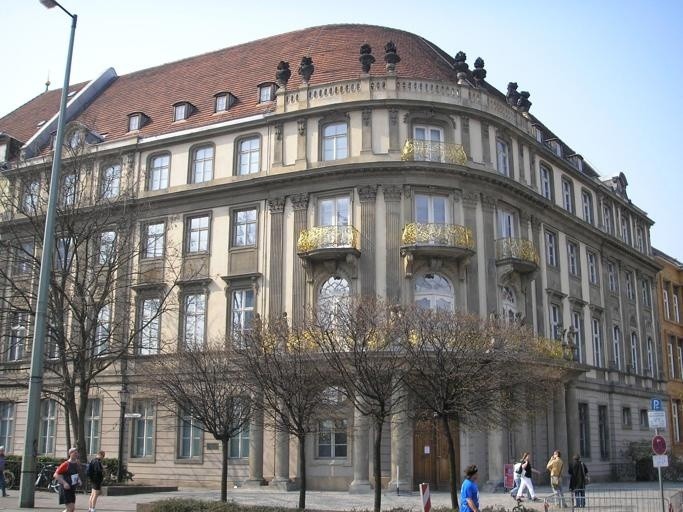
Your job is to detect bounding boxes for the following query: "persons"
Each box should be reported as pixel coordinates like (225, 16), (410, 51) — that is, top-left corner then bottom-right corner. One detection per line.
(568, 454), (589, 508)
(87, 451), (105, 512)
(53, 448), (82, 512)
(0, 446), (10, 497)
(510, 459), (525, 498)
(546, 449), (568, 507)
(517, 451), (541, 503)
(458, 464), (480, 512)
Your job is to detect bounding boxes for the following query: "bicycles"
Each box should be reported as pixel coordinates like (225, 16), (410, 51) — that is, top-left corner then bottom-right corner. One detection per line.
(35, 461), (60, 494)
(2, 468), (15, 489)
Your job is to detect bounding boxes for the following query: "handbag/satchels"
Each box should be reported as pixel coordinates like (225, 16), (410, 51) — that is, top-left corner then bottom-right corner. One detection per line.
(552, 476), (559, 486)
(516, 465), (522, 473)
(584, 473), (590, 484)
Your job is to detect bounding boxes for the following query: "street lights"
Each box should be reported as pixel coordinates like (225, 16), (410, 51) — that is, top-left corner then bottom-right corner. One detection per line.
(19, 0), (80, 508)
